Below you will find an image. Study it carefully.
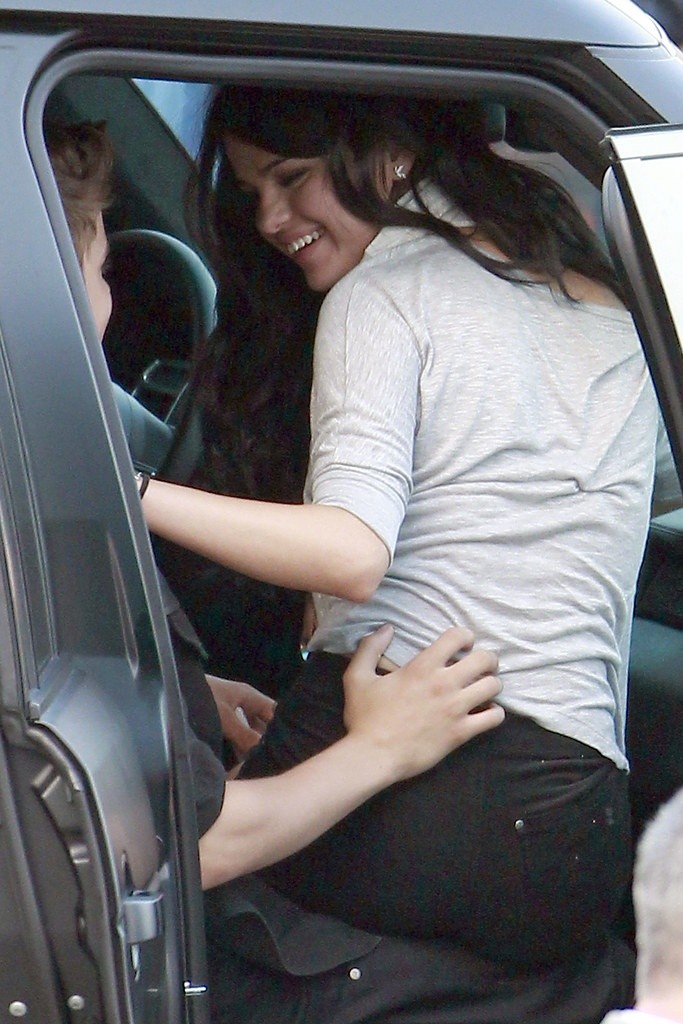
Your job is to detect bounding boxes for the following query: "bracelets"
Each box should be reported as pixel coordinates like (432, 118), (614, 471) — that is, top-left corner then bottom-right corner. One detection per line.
(137, 473), (150, 500)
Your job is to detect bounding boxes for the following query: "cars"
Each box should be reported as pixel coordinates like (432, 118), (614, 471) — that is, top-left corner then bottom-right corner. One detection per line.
(0, 0), (683, 1024)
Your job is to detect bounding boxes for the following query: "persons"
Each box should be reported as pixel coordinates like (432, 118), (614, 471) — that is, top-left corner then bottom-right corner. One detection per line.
(136, 85), (663, 963)
(42, 80), (637, 1024)
